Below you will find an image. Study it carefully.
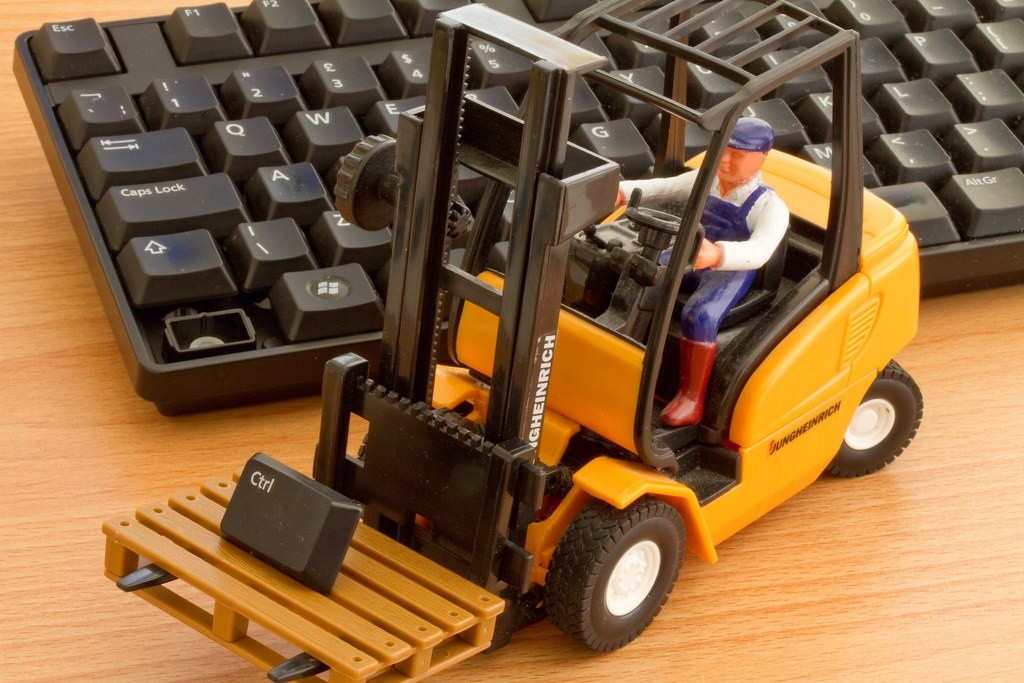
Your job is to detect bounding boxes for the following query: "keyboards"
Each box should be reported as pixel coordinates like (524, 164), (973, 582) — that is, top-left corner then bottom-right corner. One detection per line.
(12, 0), (1024, 417)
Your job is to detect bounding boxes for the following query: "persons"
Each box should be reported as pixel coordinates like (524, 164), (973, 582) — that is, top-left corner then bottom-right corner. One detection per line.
(615, 119), (789, 427)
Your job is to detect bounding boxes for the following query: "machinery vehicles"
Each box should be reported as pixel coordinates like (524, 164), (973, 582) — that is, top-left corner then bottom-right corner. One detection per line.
(94, 1), (927, 683)
